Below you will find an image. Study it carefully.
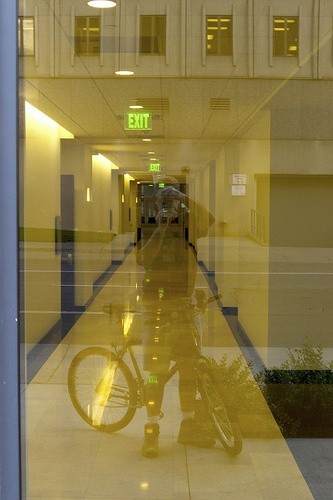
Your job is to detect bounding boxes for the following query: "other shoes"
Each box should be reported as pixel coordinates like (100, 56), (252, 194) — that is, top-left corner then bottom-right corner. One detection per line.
(177, 418), (216, 447)
(143, 423), (160, 458)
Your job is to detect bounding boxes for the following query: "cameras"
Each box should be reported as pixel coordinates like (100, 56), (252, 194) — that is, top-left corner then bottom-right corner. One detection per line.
(161, 188), (173, 224)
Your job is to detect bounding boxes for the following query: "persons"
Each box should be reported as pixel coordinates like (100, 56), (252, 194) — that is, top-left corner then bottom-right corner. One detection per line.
(134, 176), (216, 459)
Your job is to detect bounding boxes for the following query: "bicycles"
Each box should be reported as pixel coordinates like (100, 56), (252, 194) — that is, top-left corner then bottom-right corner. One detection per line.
(65, 289), (244, 460)
